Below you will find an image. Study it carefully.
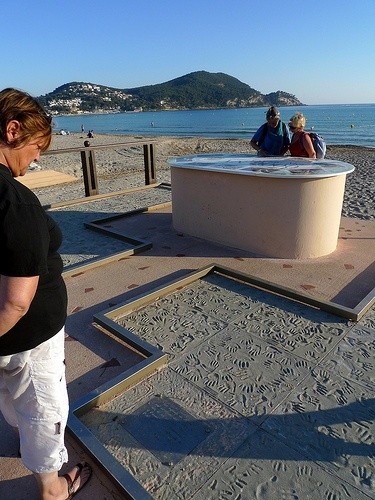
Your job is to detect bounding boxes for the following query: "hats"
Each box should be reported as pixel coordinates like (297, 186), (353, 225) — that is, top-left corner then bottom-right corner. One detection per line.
(267, 106), (280, 119)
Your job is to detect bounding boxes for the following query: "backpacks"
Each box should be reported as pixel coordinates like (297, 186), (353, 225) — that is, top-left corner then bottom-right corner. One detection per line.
(290, 132), (326, 159)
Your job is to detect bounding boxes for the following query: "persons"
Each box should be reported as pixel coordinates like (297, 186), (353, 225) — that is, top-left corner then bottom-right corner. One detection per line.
(55, 120), (154, 138)
(250, 107), (290, 156)
(288, 115), (327, 158)
(0, 88), (95, 500)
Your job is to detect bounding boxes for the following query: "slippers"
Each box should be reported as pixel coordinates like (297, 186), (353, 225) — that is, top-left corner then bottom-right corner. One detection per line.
(60, 461), (92, 500)
